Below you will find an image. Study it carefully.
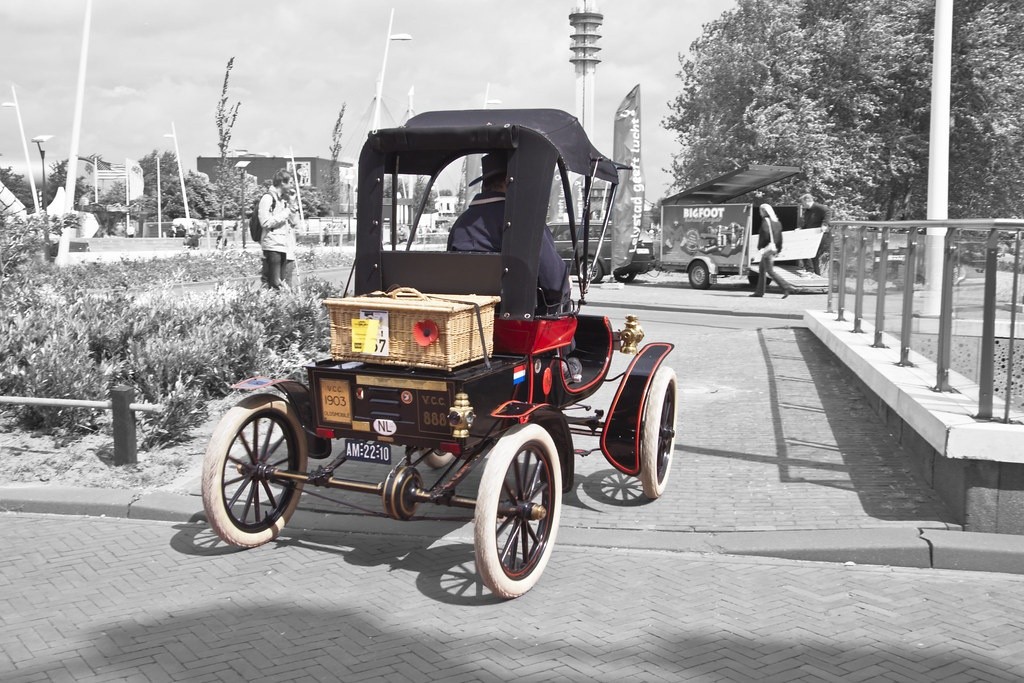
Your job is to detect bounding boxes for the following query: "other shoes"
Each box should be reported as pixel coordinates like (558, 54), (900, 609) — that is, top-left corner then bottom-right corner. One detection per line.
(782, 285), (795, 299)
(749, 293), (763, 298)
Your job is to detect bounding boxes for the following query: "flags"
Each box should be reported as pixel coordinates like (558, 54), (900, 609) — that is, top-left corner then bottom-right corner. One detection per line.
(613, 81), (646, 273)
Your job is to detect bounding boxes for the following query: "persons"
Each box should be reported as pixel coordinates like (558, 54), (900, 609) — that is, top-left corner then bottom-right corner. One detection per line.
(794, 194), (836, 277)
(749, 202), (792, 298)
(126, 223), (136, 238)
(447, 150), (583, 390)
(257, 168), (303, 289)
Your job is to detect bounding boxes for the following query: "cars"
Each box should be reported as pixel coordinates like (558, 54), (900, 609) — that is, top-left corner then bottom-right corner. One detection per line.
(831, 224), (1019, 287)
(211, 106), (679, 591)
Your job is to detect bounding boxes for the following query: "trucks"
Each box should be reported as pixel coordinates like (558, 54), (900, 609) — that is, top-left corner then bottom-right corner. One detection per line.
(649, 163), (830, 294)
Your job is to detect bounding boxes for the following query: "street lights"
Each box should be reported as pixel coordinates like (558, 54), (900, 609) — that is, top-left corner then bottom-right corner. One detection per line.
(234, 160), (250, 249)
(32, 134), (58, 259)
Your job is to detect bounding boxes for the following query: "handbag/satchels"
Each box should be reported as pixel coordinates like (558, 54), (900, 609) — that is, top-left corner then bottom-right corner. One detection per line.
(758, 241), (777, 259)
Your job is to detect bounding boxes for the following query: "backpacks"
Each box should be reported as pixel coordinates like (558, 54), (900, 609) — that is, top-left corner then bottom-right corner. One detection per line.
(249, 192), (287, 242)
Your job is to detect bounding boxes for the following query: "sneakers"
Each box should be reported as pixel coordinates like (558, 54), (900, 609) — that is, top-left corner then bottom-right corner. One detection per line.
(564, 357), (583, 385)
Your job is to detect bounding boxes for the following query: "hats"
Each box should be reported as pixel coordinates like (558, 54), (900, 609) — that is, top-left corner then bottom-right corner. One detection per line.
(468, 149), (509, 188)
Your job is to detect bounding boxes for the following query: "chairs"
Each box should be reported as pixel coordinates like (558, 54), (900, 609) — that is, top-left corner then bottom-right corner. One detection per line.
(379, 248), (578, 399)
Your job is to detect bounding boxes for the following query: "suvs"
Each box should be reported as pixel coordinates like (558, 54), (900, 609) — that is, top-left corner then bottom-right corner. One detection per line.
(543, 220), (655, 285)
